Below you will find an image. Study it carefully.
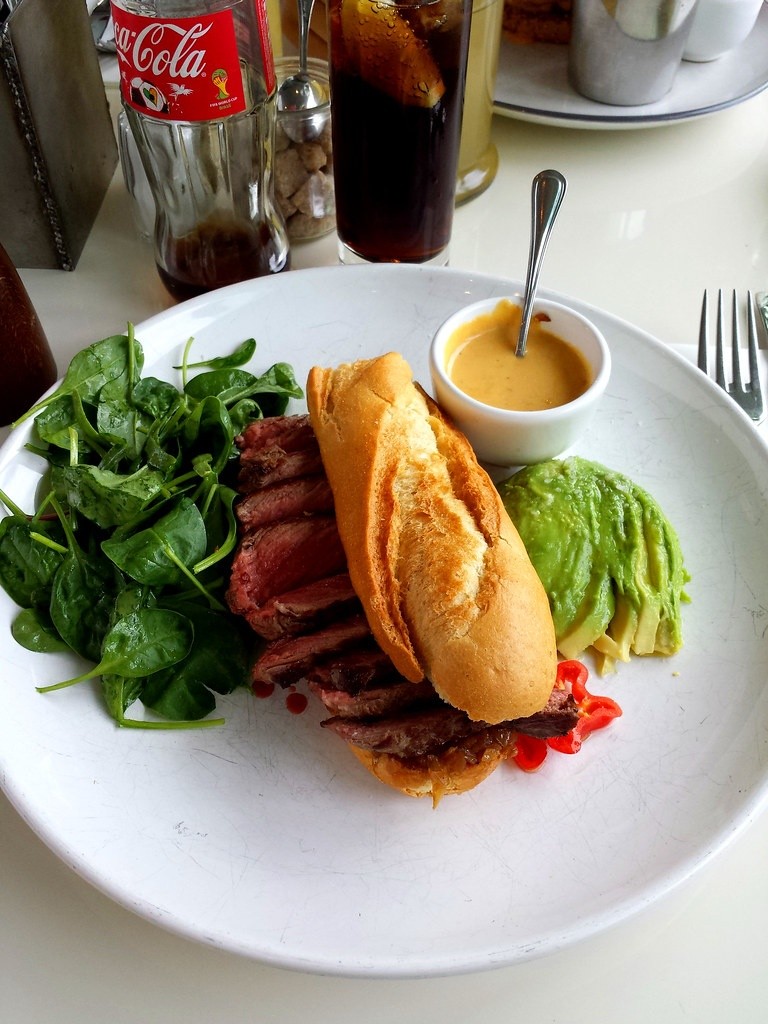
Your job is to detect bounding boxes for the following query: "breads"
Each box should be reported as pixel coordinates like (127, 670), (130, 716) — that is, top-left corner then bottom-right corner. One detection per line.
(349, 739), (502, 808)
(306, 351), (560, 724)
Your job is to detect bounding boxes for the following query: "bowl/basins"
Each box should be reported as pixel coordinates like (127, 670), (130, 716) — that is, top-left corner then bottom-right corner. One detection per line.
(678, 0), (766, 66)
(430, 293), (611, 465)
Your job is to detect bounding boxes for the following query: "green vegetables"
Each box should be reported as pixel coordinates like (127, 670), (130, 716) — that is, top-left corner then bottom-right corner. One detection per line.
(0, 321), (306, 732)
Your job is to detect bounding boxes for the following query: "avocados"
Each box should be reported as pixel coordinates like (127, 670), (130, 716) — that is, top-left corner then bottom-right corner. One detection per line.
(498, 456), (693, 676)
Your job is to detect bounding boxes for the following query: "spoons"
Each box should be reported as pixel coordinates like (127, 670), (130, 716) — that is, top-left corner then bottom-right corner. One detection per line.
(276, 1), (329, 143)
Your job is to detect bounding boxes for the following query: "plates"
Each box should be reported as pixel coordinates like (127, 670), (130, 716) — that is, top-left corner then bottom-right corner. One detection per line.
(1, 262), (767, 977)
(492, 0), (768, 130)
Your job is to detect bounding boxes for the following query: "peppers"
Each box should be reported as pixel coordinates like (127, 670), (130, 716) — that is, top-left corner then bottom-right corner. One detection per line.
(508, 659), (623, 773)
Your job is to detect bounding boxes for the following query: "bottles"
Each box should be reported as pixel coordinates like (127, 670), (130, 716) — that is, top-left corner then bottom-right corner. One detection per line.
(108, 1), (291, 304)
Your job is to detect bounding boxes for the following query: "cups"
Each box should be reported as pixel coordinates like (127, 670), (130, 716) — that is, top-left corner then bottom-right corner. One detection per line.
(269, 56), (330, 241)
(567, 0), (696, 107)
(456, 2), (504, 208)
(327, 0), (473, 265)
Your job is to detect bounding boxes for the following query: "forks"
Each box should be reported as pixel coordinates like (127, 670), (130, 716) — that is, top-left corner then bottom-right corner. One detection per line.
(697, 289), (762, 424)
